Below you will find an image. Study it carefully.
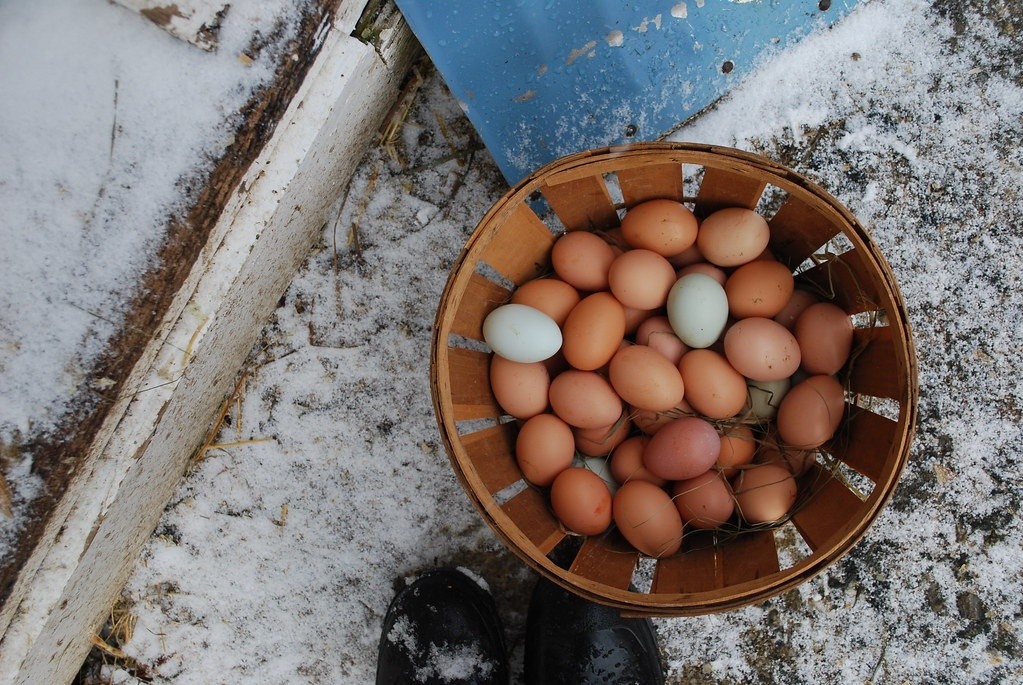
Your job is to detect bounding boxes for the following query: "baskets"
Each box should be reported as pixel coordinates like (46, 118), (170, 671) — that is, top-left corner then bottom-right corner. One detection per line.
(425, 143), (919, 619)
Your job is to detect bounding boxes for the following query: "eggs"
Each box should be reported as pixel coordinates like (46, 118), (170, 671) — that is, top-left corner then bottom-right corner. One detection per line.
(483, 198), (854, 559)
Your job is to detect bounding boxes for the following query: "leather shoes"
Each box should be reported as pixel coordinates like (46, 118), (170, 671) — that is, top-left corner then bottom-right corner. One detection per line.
(522, 584), (668, 685)
(373, 571), (509, 683)
